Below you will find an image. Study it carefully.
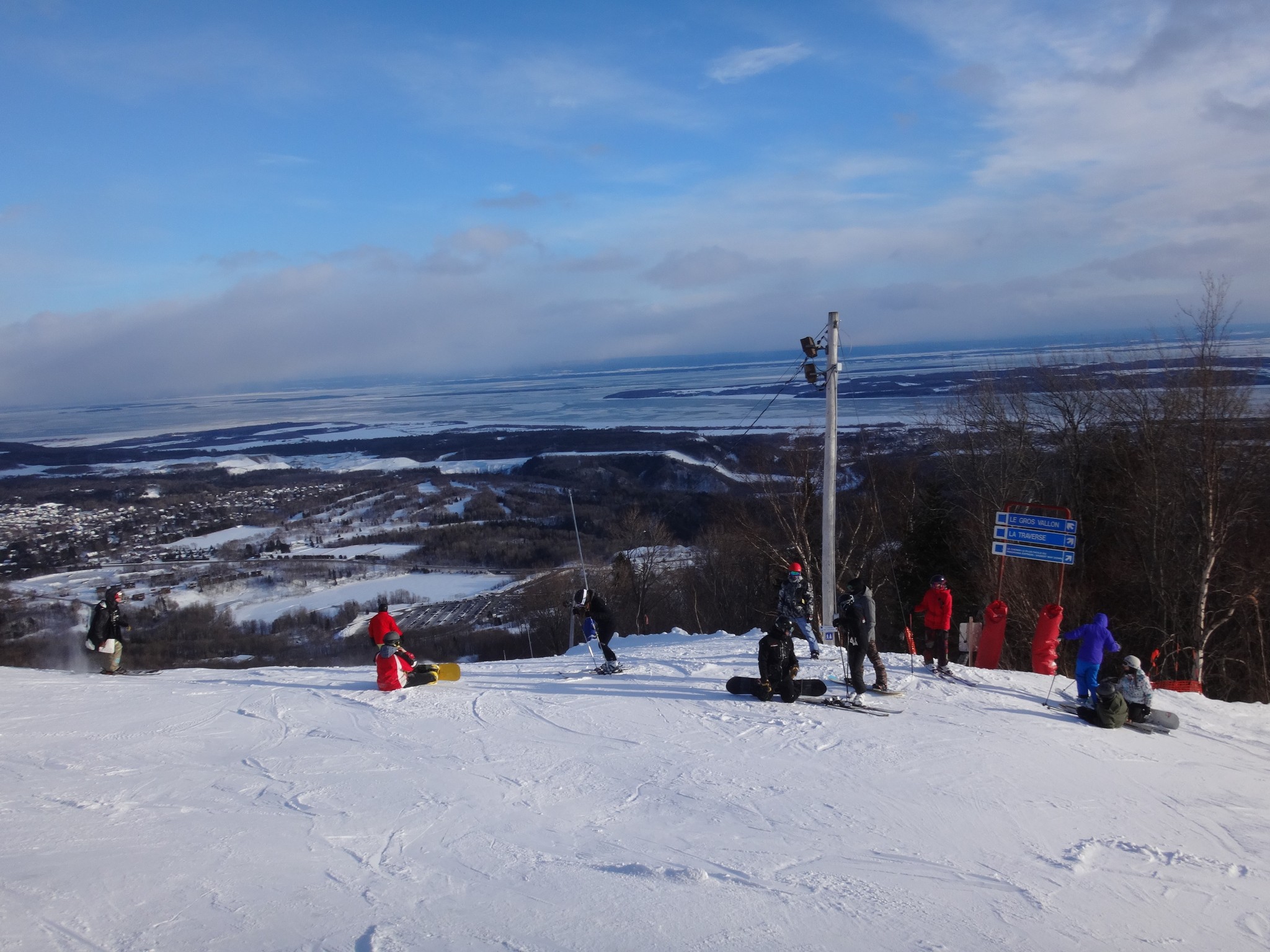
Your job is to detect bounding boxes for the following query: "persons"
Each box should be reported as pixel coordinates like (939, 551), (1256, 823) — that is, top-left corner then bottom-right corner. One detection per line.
(368, 604), (403, 650)
(832, 578), (888, 706)
(373, 631), (440, 691)
(776, 562), (820, 660)
(1078, 681), (1129, 729)
(754, 615), (802, 703)
(1114, 655), (1153, 723)
(910, 574), (953, 675)
(1057, 612), (1122, 705)
(85, 587), (128, 674)
(572, 588), (618, 673)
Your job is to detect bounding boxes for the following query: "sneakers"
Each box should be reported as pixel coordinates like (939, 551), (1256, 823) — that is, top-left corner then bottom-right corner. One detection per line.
(872, 683), (887, 690)
(853, 692), (865, 703)
(926, 664), (936, 670)
(113, 666), (126, 674)
(844, 677), (852, 684)
(810, 651), (819, 660)
(939, 665), (951, 673)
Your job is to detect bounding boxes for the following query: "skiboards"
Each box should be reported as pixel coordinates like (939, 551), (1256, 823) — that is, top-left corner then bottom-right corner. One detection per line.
(70, 669), (164, 676)
(919, 659), (978, 687)
(559, 663), (652, 678)
(796, 697), (905, 717)
(1057, 702), (1170, 736)
(1055, 687), (1088, 705)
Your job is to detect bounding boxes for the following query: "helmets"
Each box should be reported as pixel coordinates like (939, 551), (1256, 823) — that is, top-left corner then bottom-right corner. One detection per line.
(1123, 655), (1141, 670)
(1096, 683), (1116, 700)
(930, 574), (945, 587)
(775, 616), (794, 634)
(838, 594), (854, 610)
(383, 631), (400, 645)
(788, 563), (802, 572)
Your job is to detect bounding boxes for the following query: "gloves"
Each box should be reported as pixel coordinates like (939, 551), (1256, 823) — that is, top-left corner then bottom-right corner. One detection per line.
(806, 614), (812, 623)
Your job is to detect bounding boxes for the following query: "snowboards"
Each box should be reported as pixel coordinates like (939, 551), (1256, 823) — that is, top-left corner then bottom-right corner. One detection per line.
(726, 675), (828, 698)
(828, 675), (904, 695)
(1145, 708), (1180, 730)
(410, 662), (461, 682)
(795, 655), (841, 662)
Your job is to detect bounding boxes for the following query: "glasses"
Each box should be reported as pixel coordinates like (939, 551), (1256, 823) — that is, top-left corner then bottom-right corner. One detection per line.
(847, 585), (853, 590)
(790, 571), (800, 575)
(930, 583), (941, 587)
(1123, 660), (1130, 667)
(115, 591), (123, 598)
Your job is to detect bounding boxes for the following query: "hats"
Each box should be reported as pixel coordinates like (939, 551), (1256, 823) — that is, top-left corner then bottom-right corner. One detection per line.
(379, 603), (388, 612)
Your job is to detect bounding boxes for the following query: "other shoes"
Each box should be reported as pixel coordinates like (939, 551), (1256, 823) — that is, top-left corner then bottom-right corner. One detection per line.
(597, 665), (618, 675)
(594, 663), (606, 671)
